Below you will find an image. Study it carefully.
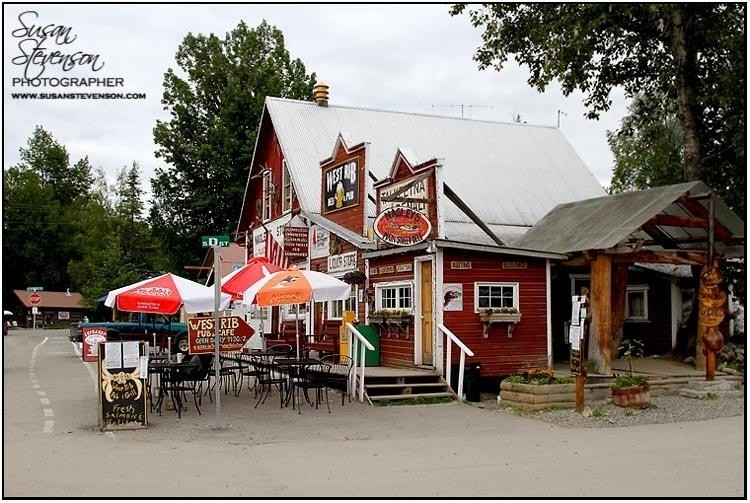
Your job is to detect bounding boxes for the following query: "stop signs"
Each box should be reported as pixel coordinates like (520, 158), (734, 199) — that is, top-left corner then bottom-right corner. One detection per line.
(29, 293), (40, 305)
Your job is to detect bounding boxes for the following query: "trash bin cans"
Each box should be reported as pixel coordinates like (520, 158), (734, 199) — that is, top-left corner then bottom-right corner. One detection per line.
(70, 323), (79, 341)
(451, 363), (481, 402)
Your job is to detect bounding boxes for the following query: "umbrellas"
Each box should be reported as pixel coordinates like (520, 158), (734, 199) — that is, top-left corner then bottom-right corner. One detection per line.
(208, 256), (288, 351)
(236, 266), (353, 404)
(95, 276), (158, 387)
(105, 270), (233, 400)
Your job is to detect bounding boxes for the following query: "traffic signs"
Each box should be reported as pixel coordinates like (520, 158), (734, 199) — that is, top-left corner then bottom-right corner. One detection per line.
(188, 317), (255, 353)
(201, 236), (230, 247)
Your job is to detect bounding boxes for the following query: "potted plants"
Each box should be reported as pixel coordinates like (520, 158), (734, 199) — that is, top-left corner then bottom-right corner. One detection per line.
(611, 338), (652, 409)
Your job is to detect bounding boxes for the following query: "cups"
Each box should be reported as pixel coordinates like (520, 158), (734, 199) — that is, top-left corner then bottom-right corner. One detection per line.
(243, 346), (248, 352)
(176, 352), (182, 362)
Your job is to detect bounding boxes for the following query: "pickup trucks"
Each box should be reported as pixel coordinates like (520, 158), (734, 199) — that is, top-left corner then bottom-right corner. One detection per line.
(68, 311), (199, 350)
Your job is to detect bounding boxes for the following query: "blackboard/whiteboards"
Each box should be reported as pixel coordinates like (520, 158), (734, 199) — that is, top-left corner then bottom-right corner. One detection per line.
(101, 343), (145, 430)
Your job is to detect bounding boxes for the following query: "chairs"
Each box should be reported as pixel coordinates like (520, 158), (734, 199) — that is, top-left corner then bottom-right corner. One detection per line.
(147, 342), (293, 419)
(315, 354), (354, 406)
(294, 362), (333, 413)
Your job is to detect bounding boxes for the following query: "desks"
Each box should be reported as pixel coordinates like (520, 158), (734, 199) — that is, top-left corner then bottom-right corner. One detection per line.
(274, 358), (323, 407)
